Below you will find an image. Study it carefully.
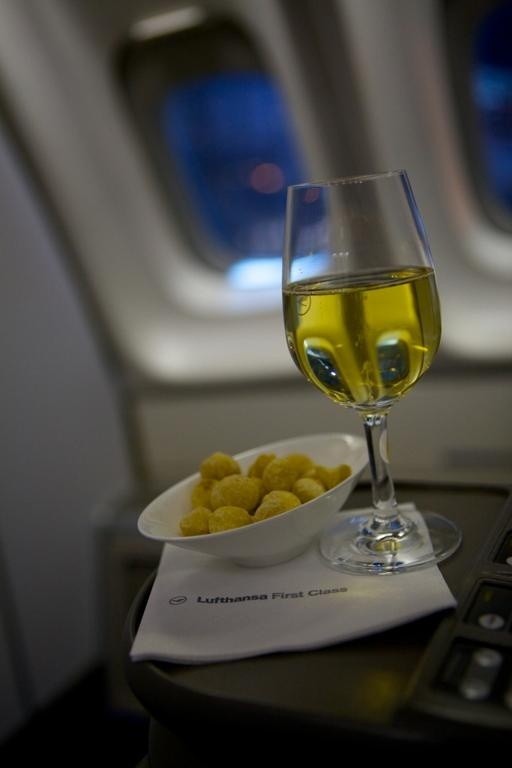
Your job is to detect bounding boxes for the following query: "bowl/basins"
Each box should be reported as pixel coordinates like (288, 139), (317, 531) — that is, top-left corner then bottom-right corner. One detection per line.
(136, 430), (369, 572)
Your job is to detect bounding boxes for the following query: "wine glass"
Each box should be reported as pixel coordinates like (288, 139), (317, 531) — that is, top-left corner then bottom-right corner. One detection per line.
(281, 166), (465, 573)
